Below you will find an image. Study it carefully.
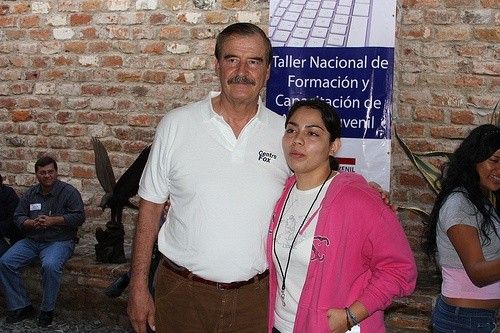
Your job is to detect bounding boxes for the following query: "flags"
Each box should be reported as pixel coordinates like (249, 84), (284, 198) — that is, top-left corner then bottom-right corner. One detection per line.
(267, 0), (397, 191)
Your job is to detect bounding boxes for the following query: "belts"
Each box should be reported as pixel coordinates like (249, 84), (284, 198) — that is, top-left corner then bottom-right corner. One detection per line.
(160, 256), (270, 291)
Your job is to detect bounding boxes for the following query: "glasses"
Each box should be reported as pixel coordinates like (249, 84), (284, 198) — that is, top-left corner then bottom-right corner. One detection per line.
(37, 170), (57, 175)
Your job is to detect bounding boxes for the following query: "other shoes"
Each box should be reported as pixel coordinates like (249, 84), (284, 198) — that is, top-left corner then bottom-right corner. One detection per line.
(38, 311), (54, 325)
(6, 305), (33, 322)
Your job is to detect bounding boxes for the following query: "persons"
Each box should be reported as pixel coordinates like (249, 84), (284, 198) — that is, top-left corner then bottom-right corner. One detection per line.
(125, 21), (400, 333)
(420, 124), (500, 333)
(0, 174), (25, 256)
(104, 221), (163, 299)
(0, 158), (86, 325)
(163, 99), (417, 333)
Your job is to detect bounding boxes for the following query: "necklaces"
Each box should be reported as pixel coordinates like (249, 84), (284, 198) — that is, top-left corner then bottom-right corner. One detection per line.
(274, 168), (332, 306)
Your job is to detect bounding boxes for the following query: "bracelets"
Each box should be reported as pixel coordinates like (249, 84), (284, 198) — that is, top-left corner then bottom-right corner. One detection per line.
(345, 306), (361, 331)
